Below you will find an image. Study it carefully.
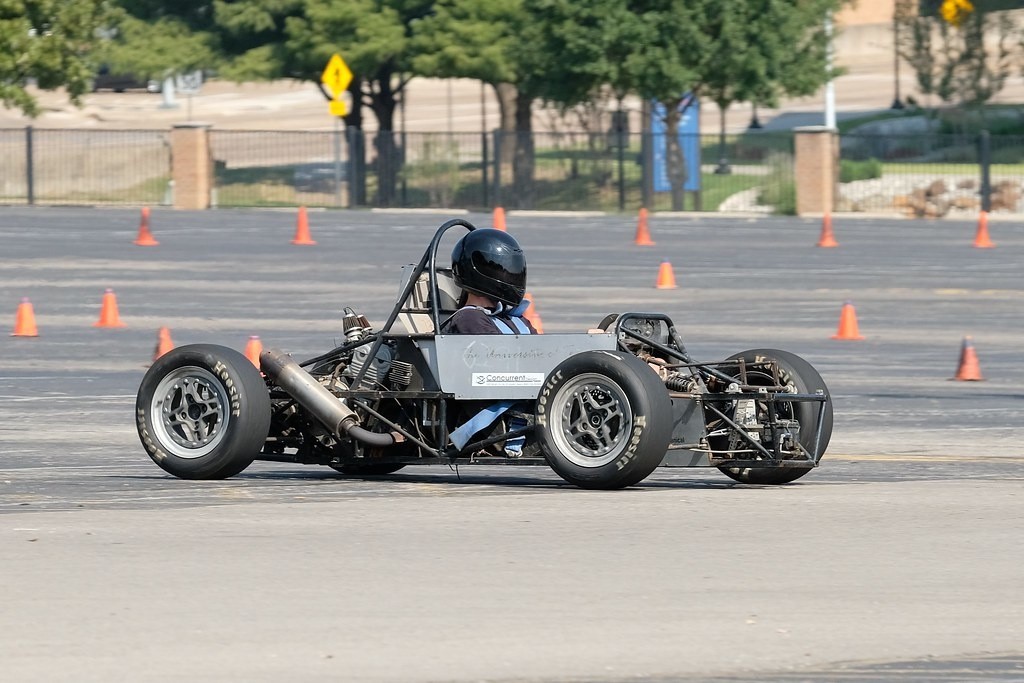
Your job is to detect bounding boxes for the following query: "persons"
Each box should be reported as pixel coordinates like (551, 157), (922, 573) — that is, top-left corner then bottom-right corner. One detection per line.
(441, 229), (540, 335)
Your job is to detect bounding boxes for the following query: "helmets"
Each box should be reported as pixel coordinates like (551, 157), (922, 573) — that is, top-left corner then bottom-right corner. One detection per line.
(451, 229), (527, 307)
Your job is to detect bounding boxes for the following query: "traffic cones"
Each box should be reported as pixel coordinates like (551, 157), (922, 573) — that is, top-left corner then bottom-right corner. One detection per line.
(244, 335), (265, 378)
(134, 207), (158, 245)
(91, 288), (128, 328)
(947, 335), (988, 382)
(493, 207), (507, 232)
(634, 207), (656, 246)
(524, 293), (544, 334)
(816, 211), (839, 248)
(652, 258), (677, 290)
(8, 297), (40, 337)
(972, 211), (995, 248)
(829, 302), (866, 340)
(288, 207), (316, 245)
(152, 327), (174, 364)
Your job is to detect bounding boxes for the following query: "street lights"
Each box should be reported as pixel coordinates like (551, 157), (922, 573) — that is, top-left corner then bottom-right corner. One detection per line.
(612, 78), (630, 211)
